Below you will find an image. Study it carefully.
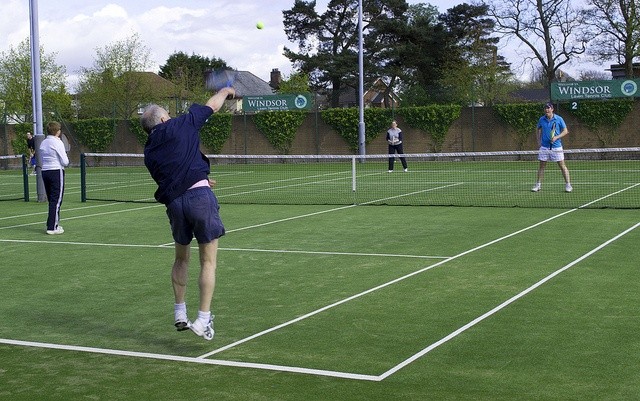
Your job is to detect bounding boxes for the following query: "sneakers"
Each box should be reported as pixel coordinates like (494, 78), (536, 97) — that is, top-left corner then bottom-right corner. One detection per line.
(531, 183), (542, 191)
(174, 311), (192, 331)
(191, 318), (215, 341)
(565, 185), (574, 191)
(46, 225), (64, 233)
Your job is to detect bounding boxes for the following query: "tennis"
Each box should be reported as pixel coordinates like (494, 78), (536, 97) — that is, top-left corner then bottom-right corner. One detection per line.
(394, 161), (396, 164)
(256, 21), (264, 29)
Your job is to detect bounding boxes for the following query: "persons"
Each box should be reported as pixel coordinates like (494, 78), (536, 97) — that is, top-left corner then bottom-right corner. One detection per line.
(39, 121), (70, 235)
(386, 121), (409, 173)
(26, 132), (37, 176)
(532, 103), (573, 193)
(141, 87), (235, 342)
